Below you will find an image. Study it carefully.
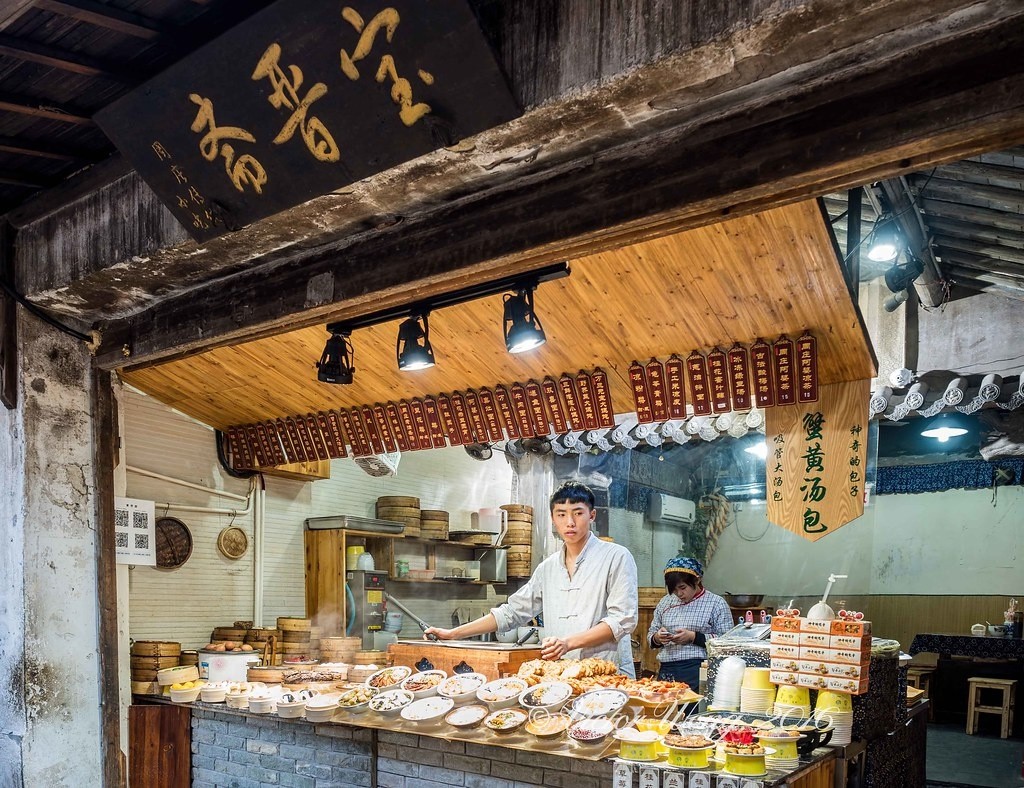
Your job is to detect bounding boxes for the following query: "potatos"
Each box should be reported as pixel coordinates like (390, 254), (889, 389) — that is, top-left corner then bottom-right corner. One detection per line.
(206, 641), (252, 652)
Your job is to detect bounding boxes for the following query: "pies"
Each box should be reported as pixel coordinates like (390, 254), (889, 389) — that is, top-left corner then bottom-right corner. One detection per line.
(517, 658), (627, 696)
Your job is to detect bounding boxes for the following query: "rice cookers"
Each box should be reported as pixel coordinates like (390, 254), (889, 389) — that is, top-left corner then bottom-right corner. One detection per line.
(196, 649), (260, 685)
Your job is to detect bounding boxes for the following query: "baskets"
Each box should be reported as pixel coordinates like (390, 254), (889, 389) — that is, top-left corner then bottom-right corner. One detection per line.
(405, 569), (436, 580)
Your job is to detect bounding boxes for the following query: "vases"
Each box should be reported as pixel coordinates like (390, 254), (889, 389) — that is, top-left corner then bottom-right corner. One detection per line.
(1003, 622), (1018, 636)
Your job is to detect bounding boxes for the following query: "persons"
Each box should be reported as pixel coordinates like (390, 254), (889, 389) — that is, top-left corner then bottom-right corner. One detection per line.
(423, 482), (638, 679)
(647, 557), (734, 715)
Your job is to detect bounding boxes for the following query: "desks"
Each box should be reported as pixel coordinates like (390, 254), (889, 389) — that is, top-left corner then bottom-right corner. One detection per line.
(910, 633), (1024, 718)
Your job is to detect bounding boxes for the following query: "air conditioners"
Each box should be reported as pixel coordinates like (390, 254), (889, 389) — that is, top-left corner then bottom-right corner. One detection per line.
(650, 493), (696, 529)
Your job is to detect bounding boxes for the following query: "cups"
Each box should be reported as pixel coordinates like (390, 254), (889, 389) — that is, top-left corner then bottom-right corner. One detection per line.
(465, 561), (481, 580)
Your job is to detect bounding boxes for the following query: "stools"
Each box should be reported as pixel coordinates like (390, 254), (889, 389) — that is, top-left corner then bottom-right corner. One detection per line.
(965, 679), (1016, 739)
(907, 671), (930, 701)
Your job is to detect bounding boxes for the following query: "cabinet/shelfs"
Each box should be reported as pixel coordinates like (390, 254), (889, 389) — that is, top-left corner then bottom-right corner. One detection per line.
(303, 529), (507, 650)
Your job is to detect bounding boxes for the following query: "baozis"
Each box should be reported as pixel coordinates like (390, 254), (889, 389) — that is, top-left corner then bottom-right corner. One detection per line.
(807, 601), (835, 620)
(170, 681), (314, 703)
(354, 663), (378, 670)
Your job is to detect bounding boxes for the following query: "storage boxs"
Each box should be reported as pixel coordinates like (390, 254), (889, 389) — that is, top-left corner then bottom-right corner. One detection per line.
(769, 616), (871, 695)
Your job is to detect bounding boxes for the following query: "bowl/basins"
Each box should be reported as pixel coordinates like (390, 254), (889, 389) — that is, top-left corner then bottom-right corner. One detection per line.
(988, 625), (1006, 636)
(366, 664), (412, 692)
(369, 688), (414, 716)
(347, 546), (376, 572)
(722, 594), (765, 608)
(739, 666), (854, 746)
(612, 719), (802, 774)
(572, 688), (630, 718)
(338, 686), (379, 713)
(525, 712), (572, 739)
(400, 669), (573, 734)
(384, 612), (405, 634)
(566, 717), (615, 743)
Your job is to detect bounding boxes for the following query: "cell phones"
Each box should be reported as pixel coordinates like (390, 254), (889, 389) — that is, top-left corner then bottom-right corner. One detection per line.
(660, 632), (674, 636)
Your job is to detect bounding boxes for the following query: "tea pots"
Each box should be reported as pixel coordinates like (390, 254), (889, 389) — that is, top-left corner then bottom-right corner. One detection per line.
(478, 507), (508, 547)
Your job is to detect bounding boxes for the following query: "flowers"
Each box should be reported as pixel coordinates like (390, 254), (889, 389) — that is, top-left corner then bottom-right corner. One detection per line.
(1003, 599), (1018, 622)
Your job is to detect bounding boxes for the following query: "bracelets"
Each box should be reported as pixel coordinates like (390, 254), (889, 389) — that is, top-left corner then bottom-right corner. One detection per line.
(653, 636), (661, 645)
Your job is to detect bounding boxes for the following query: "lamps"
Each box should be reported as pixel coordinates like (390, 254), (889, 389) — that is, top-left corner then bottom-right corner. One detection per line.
(396, 314), (435, 371)
(920, 414), (968, 443)
(503, 290), (547, 353)
(884, 259), (925, 295)
(868, 213), (902, 262)
(318, 334), (356, 386)
(882, 290), (908, 313)
